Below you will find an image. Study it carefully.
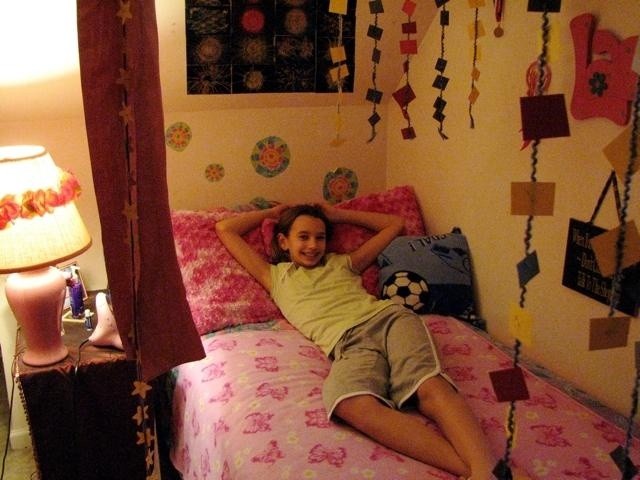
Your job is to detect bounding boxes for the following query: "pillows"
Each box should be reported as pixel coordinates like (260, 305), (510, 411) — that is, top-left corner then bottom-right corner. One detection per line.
(172, 184), (429, 334)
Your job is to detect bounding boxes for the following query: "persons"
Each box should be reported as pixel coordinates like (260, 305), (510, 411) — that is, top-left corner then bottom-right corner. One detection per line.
(214, 198), (532, 480)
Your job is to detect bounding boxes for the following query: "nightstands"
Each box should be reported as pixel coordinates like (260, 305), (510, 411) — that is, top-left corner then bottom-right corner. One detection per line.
(6, 287), (175, 479)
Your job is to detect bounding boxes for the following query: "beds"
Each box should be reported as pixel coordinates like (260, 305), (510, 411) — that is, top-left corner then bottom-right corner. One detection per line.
(162, 186), (639, 480)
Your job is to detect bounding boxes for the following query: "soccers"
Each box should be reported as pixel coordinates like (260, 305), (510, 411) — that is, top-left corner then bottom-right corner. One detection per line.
(382, 272), (433, 313)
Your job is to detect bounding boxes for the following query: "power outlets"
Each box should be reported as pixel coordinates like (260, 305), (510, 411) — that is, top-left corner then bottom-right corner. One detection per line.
(0, 142), (95, 367)
